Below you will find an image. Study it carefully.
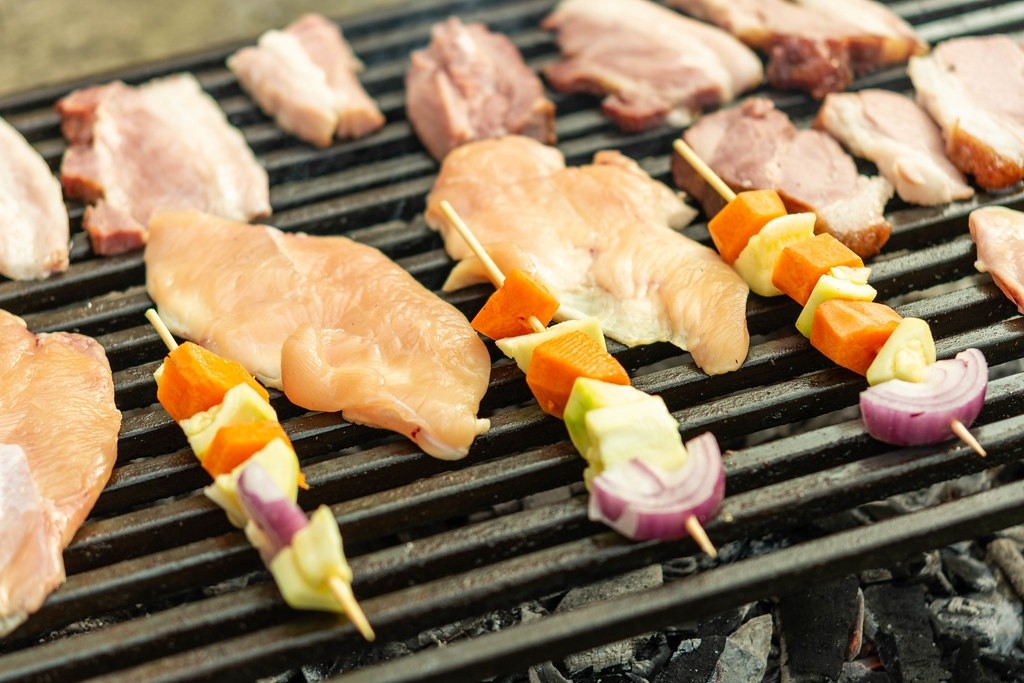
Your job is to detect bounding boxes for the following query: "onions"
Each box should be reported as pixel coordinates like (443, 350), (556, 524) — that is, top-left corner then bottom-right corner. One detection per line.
(238, 348), (987, 565)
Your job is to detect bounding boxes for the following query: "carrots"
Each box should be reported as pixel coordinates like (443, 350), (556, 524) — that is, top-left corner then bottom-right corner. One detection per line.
(155, 189), (907, 477)
